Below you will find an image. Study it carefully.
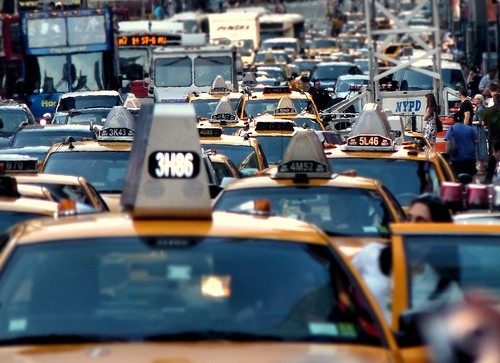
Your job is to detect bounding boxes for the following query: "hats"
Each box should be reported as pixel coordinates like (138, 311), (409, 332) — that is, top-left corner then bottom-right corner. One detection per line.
(471, 67), (480, 73)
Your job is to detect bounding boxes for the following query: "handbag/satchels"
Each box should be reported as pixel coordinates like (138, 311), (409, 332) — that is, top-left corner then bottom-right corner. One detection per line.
(436, 118), (443, 133)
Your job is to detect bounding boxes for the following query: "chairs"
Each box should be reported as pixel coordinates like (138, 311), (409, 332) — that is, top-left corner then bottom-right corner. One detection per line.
(33, 253), (100, 315)
(329, 192), (372, 227)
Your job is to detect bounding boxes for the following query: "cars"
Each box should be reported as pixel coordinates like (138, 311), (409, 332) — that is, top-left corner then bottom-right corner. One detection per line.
(247, 4), (468, 91)
(0, 73), (499, 362)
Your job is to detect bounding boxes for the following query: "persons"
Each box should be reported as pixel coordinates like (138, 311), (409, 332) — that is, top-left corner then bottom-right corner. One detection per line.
(227, 0), (349, 38)
(423, 62), (500, 189)
(93, 161), (108, 183)
(351, 195), (463, 332)
(309, 80), (330, 112)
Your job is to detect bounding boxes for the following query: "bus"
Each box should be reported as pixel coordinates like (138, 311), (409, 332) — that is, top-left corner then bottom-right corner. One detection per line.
(0, 7), (305, 101)
